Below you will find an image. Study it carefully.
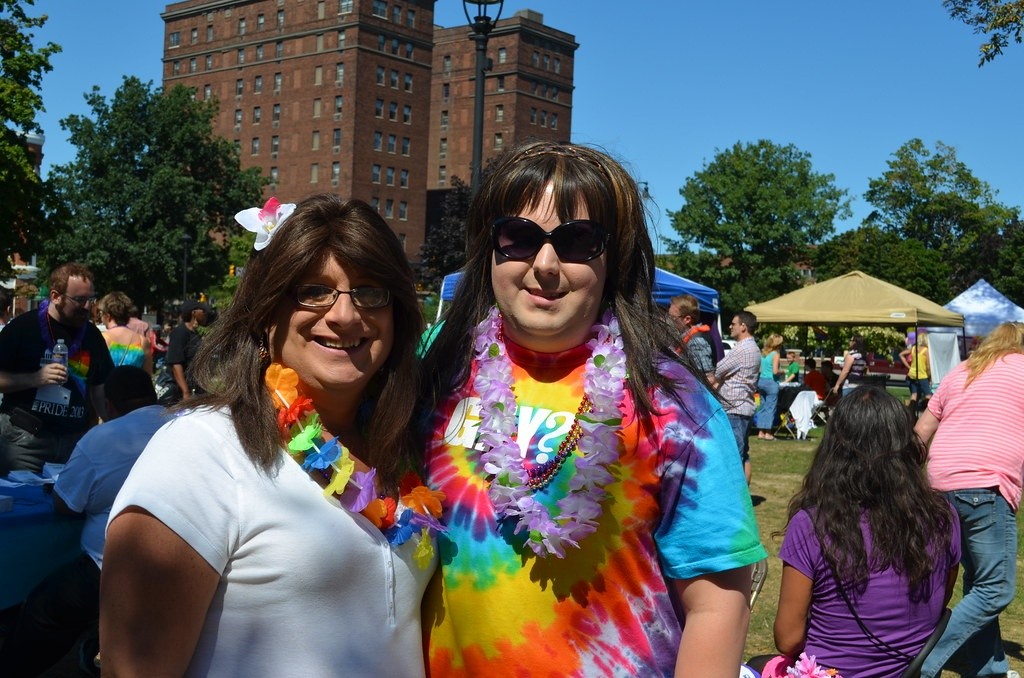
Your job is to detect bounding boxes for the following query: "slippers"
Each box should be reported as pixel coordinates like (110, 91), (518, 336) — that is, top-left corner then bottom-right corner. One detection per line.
(764, 436), (777, 441)
(757, 437), (764, 440)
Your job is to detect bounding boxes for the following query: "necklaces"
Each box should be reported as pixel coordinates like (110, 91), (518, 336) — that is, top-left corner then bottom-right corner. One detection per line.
(40, 298), (88, 361)
(471, 306), (627, 562)
(676, 325), (710, 352)
(266, 365), (444, 565)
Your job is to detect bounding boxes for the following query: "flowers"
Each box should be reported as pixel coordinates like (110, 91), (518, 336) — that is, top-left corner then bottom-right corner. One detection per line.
(235, 197), (296, 250)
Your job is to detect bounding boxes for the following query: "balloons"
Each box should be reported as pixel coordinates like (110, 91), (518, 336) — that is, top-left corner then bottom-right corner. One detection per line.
(906, 326), (924, 345)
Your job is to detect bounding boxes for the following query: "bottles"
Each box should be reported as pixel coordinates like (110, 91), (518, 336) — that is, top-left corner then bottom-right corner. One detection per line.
(52, 338), (68, 384)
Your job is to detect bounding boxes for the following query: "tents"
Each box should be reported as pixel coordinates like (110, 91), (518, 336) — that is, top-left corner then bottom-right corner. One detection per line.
(742, 269), (967, 360)
(434, 263), (722, 340)
(922, 278), (1024, 337)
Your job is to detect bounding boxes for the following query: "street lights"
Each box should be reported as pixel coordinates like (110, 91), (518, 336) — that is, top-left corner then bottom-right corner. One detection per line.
(463, 0), (504, 209)
(181, 234), (192, 302)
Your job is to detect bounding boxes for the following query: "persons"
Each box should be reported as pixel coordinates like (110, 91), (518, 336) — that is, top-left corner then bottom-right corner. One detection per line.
(411, 138), (768, 678)
(669, 294), (714, 385)
(715, 311), (762, 486)
(100, 191), (445, 678)
(0, 364), (167, 678)
(898, 329), (931, 404)
(802, 359), (840, 408)
(913, 321), (1024, 678)
(0, 263), (212, 477)
(755, 334), (785, 440)
(835, 334), (866, 399)
(746, 385), (961, 678)
(785, 352), (799, 382)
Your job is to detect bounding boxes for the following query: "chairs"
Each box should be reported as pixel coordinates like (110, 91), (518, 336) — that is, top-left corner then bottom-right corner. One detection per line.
(816, 388), (834, 426)
(776, 390), (815, 440)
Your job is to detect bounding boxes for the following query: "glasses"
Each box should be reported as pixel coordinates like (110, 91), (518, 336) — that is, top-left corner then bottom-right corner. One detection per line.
(284, 285), (393, 310)
(850, 339), (854, 342)
(491, 216), (611, 262)
(58, 290), (97, 309)
(668, 314), (682, 321)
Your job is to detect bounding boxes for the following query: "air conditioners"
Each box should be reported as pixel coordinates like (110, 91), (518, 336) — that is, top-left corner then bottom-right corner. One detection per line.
(330, 179), (339, 186)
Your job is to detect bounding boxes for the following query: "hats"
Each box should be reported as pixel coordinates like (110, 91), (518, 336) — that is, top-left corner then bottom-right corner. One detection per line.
(180, 301), (208, 315)
(908, 332), (915, 339)
